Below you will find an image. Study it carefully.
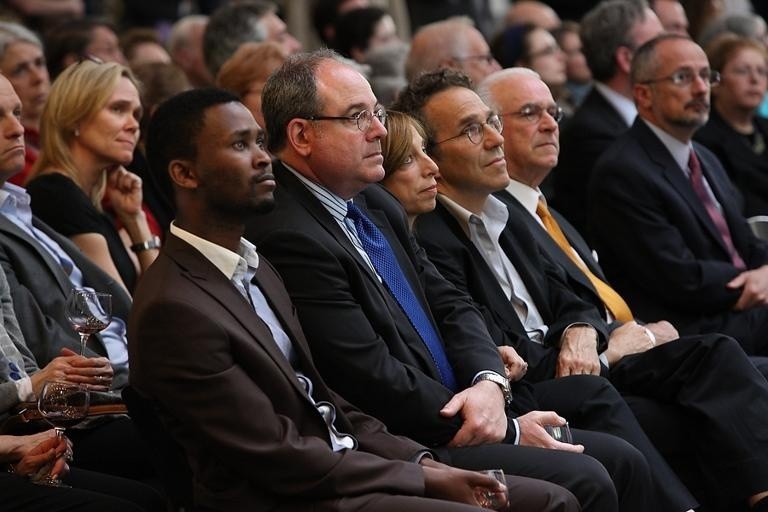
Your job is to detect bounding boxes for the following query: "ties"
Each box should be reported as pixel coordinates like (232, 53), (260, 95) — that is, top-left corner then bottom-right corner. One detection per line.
(687, 147), (747, 271)
(535, 198), (635, 324)
(344, 199), (459, 394)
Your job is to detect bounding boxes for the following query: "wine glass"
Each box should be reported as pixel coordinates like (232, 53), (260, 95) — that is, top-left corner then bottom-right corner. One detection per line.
(32, 380), (90, 487)
(63, 287), (114, 355)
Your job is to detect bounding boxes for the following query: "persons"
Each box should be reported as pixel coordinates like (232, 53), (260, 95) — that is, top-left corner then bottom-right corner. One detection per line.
(1, 1), (768, 512)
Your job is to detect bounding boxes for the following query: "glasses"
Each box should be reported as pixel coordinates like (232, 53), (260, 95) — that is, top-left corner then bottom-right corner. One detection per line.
(305, 106), (388, 134)
(429, 114), (504, 146)
(66, 51), (104, 82)
(640, 68), (722, 89)
(500, 104), (564, 125)
(529, 44), (562, 59)
(457, 50), (495, 65)
